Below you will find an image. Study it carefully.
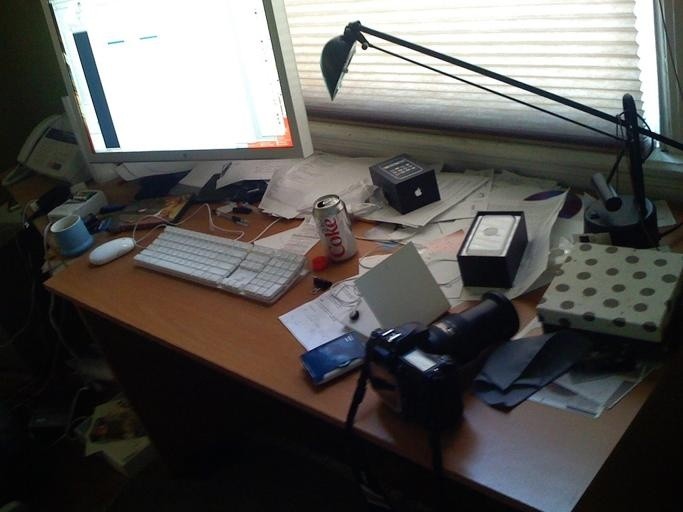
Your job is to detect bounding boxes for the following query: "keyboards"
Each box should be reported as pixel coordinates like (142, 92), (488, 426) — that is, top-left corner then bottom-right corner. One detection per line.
(133, 226), (311, 305)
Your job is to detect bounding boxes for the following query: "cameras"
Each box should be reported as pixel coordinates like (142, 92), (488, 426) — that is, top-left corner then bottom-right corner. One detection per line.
(365, 290), (520, 438)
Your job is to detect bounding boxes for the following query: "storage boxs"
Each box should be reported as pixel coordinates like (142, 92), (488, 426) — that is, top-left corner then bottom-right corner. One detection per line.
(535, 240), (683, 361)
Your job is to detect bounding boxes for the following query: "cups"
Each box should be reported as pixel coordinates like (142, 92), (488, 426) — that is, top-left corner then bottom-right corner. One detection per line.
(49, 214), (94, 259)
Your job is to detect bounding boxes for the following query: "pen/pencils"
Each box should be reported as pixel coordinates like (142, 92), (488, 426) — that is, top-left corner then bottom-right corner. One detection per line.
(213, 208), (249, 227)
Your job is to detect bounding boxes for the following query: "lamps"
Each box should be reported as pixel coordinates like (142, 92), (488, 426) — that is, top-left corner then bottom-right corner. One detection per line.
(319, 20), (682, 248)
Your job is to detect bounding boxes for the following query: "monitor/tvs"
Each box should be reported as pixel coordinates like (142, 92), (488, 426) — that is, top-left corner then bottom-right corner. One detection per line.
(31, 0), (315, 196)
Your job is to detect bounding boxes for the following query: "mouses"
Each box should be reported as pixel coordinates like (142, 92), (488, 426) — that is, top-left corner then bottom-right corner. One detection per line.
(89, 237), (136, 266)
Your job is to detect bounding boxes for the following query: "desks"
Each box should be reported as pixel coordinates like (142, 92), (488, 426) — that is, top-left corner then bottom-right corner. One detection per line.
(41, 154), (683, 511)
(0, 152), (151, 270)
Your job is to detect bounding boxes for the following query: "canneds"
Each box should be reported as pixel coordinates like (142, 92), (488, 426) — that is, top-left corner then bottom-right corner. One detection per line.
(312, 194), (358, 261)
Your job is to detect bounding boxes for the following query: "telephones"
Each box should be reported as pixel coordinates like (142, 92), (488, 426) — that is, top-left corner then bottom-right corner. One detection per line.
(1, 112), (85, 187)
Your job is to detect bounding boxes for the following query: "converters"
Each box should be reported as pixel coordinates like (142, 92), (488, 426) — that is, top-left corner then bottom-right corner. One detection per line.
(27, 411), (68, 436)
